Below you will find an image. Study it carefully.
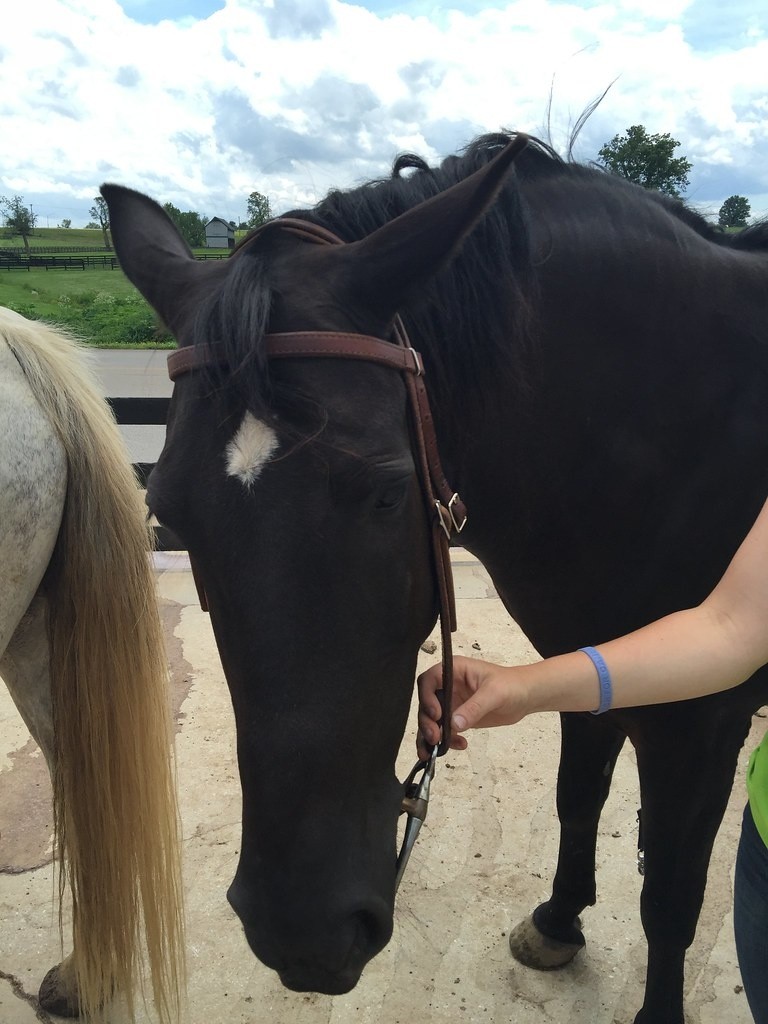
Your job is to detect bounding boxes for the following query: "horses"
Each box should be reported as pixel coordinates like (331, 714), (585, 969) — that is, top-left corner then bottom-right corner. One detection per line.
(0, 304), (193, 1015)
(95, 136), (765, 1024)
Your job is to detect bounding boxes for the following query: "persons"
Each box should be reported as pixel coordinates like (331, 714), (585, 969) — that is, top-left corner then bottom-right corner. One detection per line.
(417, 498), (768, 1024)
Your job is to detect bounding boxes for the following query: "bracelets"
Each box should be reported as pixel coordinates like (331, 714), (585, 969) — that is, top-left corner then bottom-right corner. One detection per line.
(578, 647), (611, 715)
